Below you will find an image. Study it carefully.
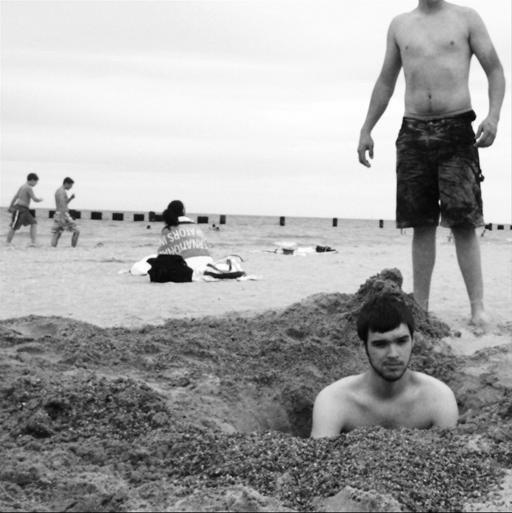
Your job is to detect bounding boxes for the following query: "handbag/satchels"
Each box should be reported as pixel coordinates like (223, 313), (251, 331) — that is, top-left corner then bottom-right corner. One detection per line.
(147, 254), (193, 282)
(204, 255), (245, 282)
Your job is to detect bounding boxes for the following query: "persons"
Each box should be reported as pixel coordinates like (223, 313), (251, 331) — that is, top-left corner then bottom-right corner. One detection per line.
(310, 294), (460, 440)
(358, 1), (506, 326)
(158, 200), (214, 281)
(50, 177), (80, 248)
(5, 173), (43, 244)
